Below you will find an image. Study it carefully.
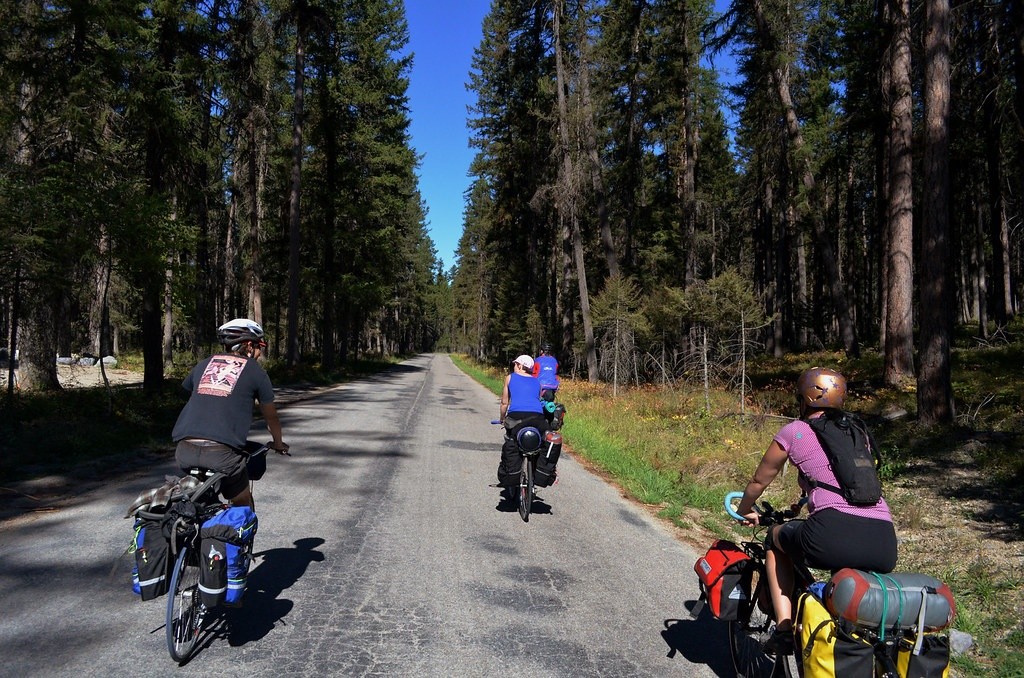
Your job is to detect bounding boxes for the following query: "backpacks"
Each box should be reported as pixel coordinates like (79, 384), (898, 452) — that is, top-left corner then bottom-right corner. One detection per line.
(800, 411), (882, 508)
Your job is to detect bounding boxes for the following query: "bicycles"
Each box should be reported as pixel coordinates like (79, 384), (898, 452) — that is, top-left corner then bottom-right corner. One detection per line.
(490, 418), (548, 522)
(123, 442), (294, 662)
(723, 491), (954, 678)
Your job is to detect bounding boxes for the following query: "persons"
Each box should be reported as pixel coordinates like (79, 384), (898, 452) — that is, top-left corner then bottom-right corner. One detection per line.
(736, 367), (898, 654)
(171, 319), (290, 610)
(533, 344), (559, 402)
(499, 355), (545, 509)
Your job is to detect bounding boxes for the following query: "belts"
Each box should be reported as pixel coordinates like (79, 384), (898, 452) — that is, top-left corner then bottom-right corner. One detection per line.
(179, 440), (231, 451)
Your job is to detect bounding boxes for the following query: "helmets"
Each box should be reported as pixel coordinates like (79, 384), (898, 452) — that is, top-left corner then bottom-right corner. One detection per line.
(218, 318), (264, 345)
(795, 366), (847, 409)
(540, 342), (552, 351)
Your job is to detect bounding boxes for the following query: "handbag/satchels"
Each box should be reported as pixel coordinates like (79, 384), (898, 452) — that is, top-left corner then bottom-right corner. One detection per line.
(551, 404), (566, 432)
(200, 505), (258, 615)
(128, 503), (183, 602)
(792, 581), (876, 678)
(694, 540), (754, 621)
(498, 437), (523, 487)
(874, 627), (949, 678)
(533, 433), (562, 488)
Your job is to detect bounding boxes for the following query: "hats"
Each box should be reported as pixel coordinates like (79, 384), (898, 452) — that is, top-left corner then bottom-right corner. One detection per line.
(251, 339), (266, 349)
(512, 354), (535, 369)
